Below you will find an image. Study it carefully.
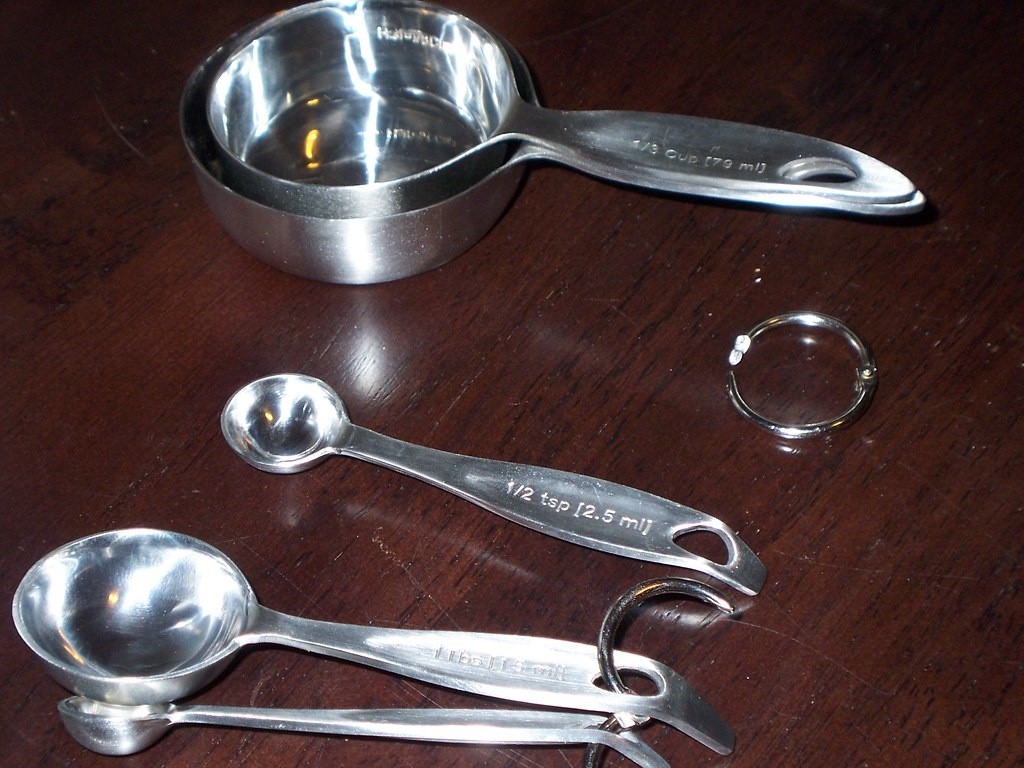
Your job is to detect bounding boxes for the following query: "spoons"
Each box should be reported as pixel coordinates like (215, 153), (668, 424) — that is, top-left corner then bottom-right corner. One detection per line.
(220, 373), (769, 598)
(11, 527), (737, 756)
(52, 694), (674, 768)
